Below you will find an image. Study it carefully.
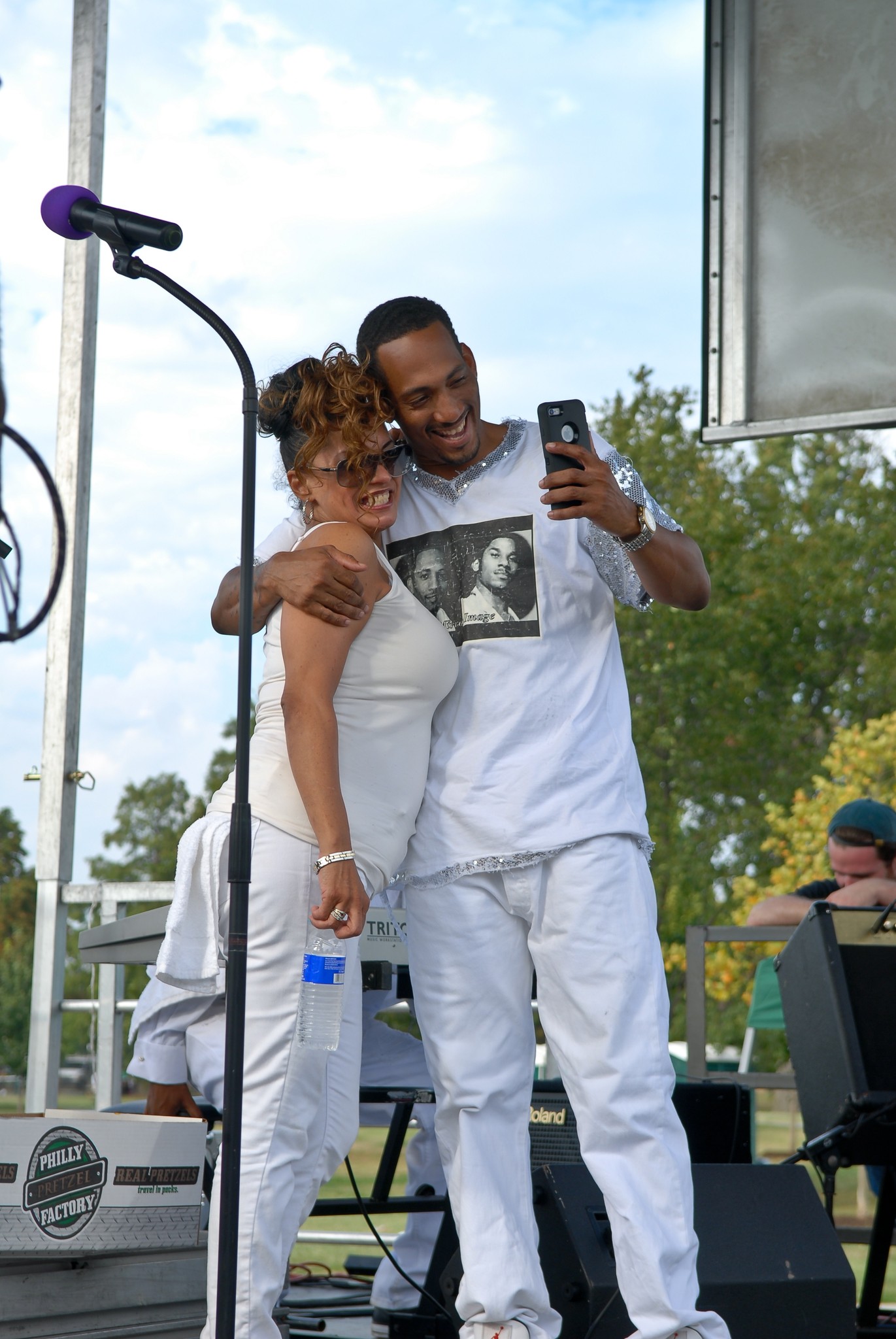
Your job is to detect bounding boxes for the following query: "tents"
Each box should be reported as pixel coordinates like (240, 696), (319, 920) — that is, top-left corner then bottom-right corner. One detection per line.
(533, 955), (786, 1166)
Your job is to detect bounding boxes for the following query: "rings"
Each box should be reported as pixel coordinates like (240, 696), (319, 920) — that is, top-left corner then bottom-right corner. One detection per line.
(328, 908), (350, 922)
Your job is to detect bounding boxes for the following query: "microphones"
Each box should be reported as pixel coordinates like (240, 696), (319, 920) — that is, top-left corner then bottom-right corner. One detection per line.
(41, 185), (183, 251)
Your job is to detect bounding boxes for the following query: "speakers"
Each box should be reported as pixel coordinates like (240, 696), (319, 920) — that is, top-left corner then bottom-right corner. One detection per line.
(772, 901), (896, 1167)
(415, 1078), (858, 1338)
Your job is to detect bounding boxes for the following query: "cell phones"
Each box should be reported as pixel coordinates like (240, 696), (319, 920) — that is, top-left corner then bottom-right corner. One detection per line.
(537, 399), (593, 511)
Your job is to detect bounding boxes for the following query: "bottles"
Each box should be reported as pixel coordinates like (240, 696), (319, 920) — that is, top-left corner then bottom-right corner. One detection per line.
(296, 929), (346, 1051)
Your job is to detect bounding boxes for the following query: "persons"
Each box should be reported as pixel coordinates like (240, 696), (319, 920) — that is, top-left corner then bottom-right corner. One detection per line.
(124, 293), (734, 1338)
(744, 796), (896, 1206)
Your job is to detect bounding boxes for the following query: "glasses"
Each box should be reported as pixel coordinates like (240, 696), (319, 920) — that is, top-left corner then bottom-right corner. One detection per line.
(305, 440), (407, 489)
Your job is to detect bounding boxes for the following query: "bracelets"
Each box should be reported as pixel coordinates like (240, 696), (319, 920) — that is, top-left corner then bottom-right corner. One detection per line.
(314, 849), (356, 874)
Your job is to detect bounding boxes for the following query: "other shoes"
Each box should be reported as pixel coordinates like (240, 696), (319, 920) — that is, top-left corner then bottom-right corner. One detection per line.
(370, 1306), (418, 1338)
(472, 1316), (528, 1339)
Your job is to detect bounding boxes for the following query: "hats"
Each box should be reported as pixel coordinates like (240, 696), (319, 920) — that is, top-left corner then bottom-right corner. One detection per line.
(827, 799), (896, 850)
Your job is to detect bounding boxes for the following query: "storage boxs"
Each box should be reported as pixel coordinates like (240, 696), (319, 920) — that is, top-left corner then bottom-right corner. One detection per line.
(0, 1108), (208, 1252)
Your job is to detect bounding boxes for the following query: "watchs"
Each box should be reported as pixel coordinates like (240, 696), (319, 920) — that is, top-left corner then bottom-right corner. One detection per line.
(609, 500), (656, 553)
